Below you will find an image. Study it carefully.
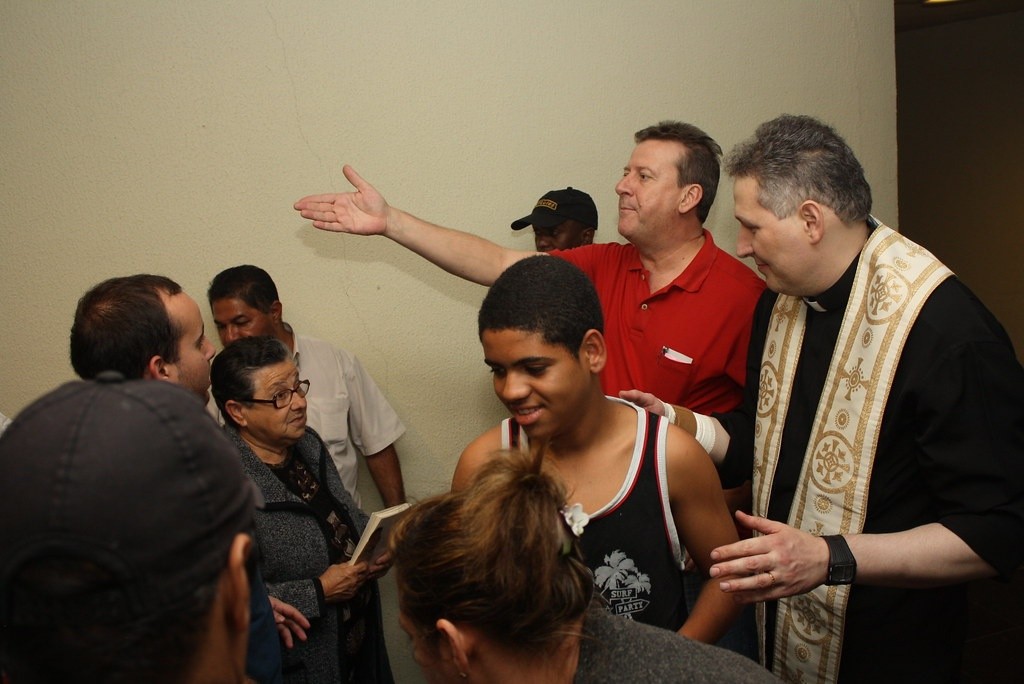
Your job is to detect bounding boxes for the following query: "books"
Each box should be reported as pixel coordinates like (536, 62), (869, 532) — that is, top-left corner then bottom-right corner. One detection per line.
(350, 503), (411, 566)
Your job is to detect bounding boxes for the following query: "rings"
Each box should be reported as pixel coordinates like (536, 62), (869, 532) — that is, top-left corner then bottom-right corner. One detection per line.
(767, 572), (775, 586)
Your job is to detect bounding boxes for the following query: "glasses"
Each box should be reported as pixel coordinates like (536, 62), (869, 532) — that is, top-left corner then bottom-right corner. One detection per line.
(239, 380), (311, 409)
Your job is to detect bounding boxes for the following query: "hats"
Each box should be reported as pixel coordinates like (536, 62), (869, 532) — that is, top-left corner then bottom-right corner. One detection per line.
(511, 187), (598, 230)
(1, 372), (251, 663)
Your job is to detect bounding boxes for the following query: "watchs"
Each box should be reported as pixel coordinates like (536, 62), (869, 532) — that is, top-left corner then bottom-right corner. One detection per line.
(819, 534), (856, 586)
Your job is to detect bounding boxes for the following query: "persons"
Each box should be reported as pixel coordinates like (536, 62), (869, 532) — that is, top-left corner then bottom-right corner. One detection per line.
(454, 254), (742, 644)
(71, 274), (310, 684)
(211, 337), (396, 683)
(1, 371), (266, 683)
(208, 266), (406, 507)
(292, 121), (767, 657)
(396, 454), (784, 684)
(511, 187), (598, 252)
(620, 115), (1024, 684)
